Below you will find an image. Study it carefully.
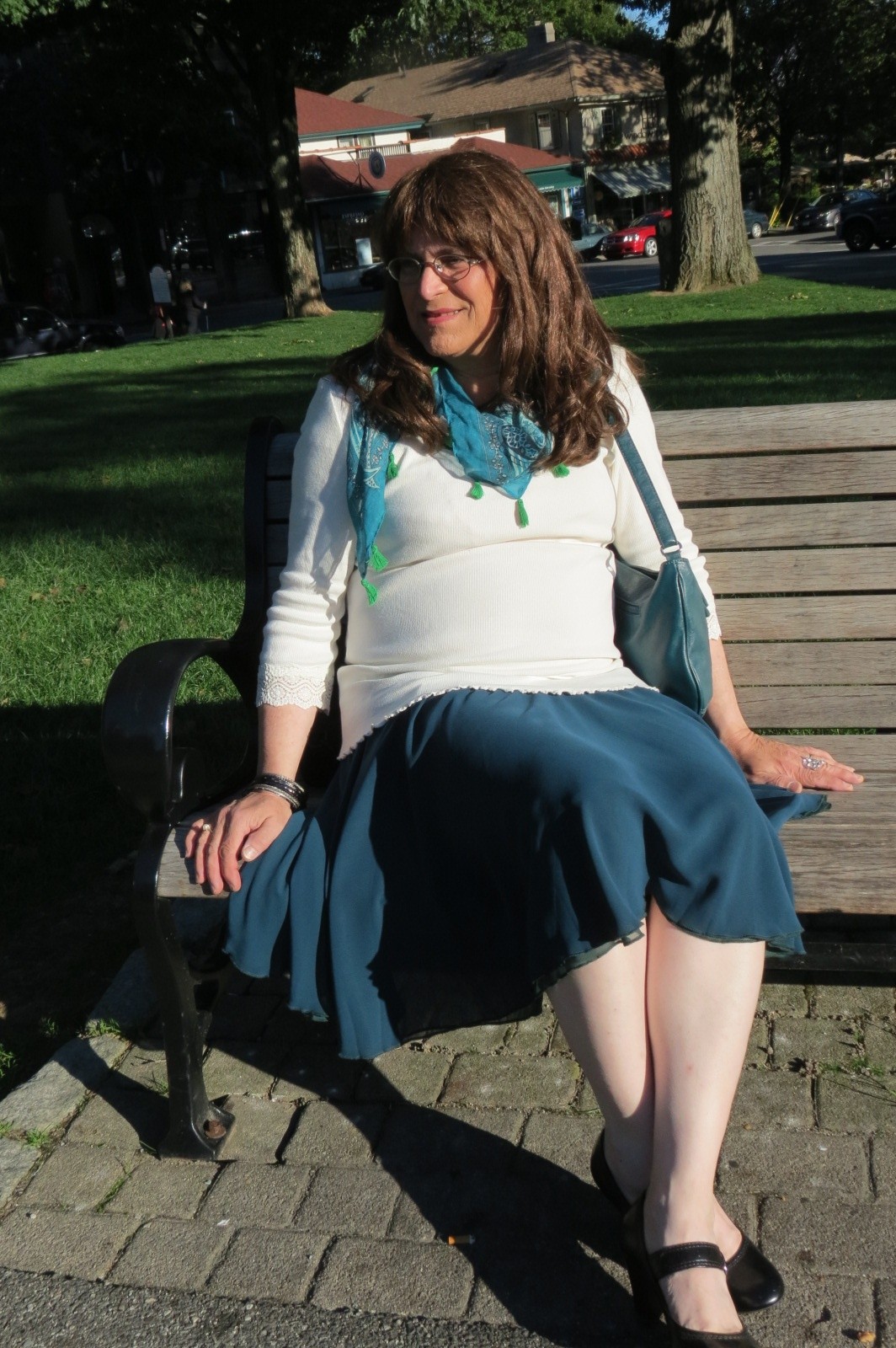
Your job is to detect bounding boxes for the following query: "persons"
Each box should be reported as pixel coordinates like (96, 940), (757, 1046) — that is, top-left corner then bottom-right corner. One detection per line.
(184, 150), (865, 1348)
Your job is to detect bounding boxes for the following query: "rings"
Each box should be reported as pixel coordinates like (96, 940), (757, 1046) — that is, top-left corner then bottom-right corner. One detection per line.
(803, 756), (826, 769)
(202, 823), (214, 832)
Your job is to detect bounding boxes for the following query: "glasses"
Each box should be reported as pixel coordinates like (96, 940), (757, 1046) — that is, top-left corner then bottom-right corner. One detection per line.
(386, 253), (492, 283)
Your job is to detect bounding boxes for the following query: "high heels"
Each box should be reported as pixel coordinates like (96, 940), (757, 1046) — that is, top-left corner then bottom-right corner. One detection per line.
(623, 1189), (760, 1348)
(591, 1128), (784, 1310)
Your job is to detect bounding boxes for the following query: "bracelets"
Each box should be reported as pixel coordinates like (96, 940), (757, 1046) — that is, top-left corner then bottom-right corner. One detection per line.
(246, 773), (304, 810)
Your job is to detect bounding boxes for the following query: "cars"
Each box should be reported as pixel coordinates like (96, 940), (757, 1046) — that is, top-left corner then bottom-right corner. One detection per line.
(601, 210), (673, 260)
(793, 190), (878, 234)
(0, 305), (127, 359)
(835, 184), (896, 252)
(742, 194), (769, 238)
(559, 216), (610, 258)
(237, 230), (265, 266)
(171, 238), (213, 271)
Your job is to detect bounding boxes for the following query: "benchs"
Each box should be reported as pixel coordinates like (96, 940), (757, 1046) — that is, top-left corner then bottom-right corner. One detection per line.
(100, 398), (896, 1163)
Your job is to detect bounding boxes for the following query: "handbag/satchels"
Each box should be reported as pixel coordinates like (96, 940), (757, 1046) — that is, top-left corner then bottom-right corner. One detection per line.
(609, 544), (713, 717)
